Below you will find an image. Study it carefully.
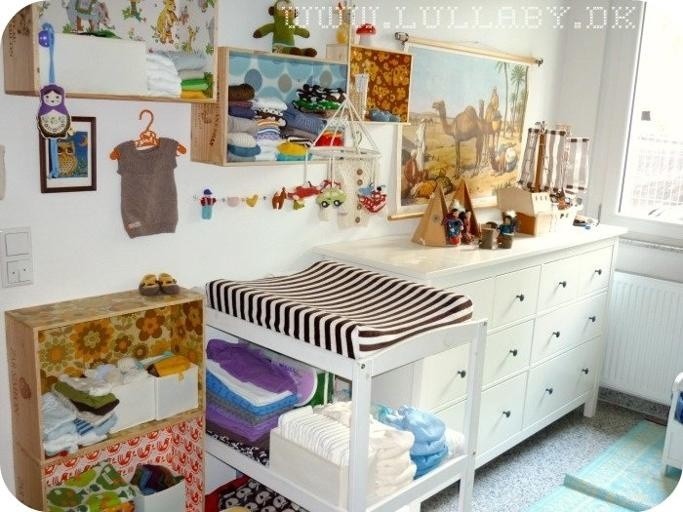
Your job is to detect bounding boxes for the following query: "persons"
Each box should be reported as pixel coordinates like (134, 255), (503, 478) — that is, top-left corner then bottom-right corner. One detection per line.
(485, 88), (502, 133)
(433, 166), (453, 194)
(405, 149), (427, 186)
(412, 115), (429, 173)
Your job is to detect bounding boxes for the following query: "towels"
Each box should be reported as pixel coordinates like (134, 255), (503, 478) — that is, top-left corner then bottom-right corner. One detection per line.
(207, 337), (300, 454)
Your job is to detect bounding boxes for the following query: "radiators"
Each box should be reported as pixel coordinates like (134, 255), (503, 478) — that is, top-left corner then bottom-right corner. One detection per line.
(602, 269), (683, 406)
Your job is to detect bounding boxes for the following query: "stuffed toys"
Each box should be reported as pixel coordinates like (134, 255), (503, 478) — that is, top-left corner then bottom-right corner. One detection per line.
(412, 179), (519, 250)
(252, 1), (316, 57)
(497, 118), (592, 238)
(285, 93), (387, 214)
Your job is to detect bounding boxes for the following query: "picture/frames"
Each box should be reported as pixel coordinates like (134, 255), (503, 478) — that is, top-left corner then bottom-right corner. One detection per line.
(38, 114), (97, 193)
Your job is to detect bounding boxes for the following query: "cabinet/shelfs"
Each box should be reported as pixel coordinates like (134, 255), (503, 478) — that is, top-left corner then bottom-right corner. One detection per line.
(191, 47), (350, 168)
(318, 224), (629, 475)
(4, 288), (206, 512)
(202, 304), (488, 510)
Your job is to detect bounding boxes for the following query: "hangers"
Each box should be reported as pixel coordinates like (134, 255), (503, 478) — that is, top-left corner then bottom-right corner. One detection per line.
(109, 109), (187, 161)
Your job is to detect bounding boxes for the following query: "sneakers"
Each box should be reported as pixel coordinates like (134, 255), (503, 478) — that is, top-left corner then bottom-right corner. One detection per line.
(158, 272), (180, 295)
(138, 273), (160, 297)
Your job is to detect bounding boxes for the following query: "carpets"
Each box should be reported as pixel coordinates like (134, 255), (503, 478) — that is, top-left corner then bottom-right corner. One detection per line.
(519, 418), (680, 512)
